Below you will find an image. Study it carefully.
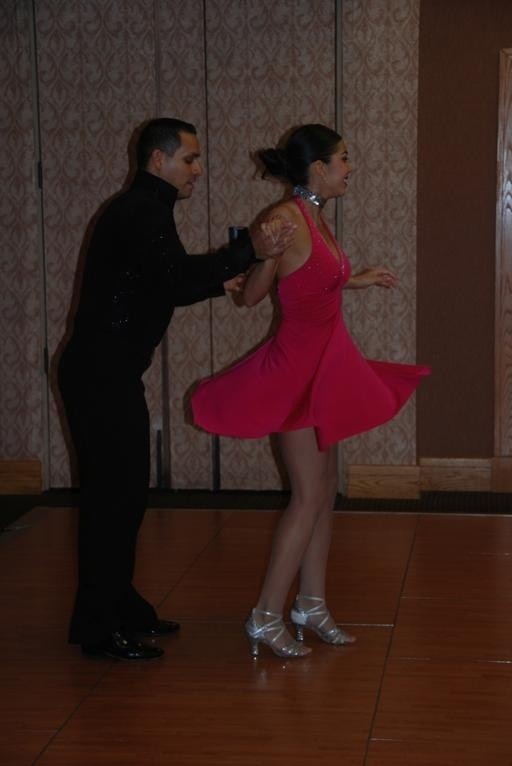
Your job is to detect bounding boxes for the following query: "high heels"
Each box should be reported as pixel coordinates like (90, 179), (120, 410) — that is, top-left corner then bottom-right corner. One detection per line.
(245, 608), (313, 658)
(290, 592), (357, 645)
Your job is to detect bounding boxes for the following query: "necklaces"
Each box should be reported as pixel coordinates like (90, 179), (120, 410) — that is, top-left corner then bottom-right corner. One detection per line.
(291, 183), (327, 211)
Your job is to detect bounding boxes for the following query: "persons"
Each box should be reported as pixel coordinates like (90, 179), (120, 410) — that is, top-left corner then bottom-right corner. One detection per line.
(187, 123), (433, 663)
(49, 118), (297, 662)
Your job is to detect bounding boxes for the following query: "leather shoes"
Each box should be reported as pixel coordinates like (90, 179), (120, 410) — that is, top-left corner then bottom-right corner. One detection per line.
(80, 620), (180, 661)
